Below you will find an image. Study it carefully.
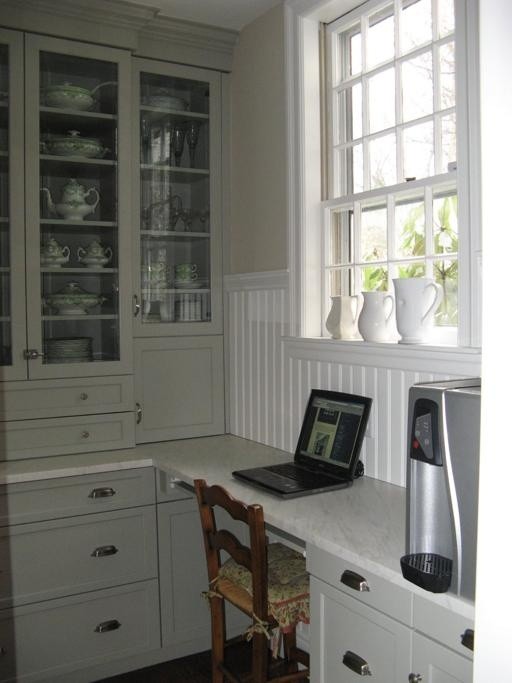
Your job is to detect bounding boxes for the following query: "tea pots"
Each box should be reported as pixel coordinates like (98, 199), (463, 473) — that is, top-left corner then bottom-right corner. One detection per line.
(46, 80), (118, 110)
(44, 283), (108, 314)
(76, 240), (112, 268)
(43, 129), (111, 160)
(41, 175), (100, 220)
(40, 237), (70, 269)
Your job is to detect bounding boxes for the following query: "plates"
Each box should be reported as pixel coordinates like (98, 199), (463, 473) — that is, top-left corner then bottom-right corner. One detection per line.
(46, 337), (94, 363)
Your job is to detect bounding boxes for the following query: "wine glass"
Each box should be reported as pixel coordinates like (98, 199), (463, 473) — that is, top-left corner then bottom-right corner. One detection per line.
(141, 118), (200, 168)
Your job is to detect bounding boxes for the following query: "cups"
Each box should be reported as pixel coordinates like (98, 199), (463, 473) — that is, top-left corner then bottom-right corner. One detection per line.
(142, 293), (209, 321)
(141, 263), (200, 289)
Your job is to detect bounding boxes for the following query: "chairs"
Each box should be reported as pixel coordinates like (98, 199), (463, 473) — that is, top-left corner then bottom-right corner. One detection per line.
(192, 475), (309, 683)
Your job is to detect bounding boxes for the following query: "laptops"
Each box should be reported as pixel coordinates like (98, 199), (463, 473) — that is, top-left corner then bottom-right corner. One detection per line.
(231, 387), (374, 500)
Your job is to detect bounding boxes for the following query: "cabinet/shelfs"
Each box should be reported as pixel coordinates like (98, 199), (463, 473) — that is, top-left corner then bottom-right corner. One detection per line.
(133, 57), (227, 446)
(304, 540), (475, 683)
(0, 28), (138, 463)
(1, 467), (158, 683)
(157, 470), (268, 660)
